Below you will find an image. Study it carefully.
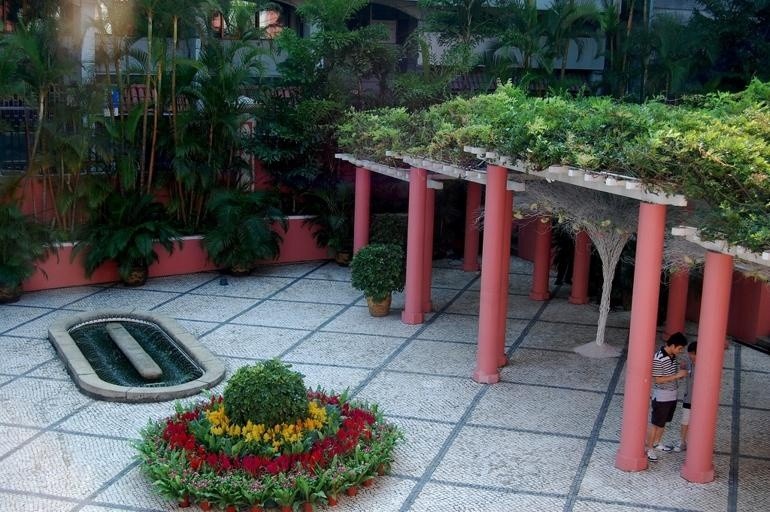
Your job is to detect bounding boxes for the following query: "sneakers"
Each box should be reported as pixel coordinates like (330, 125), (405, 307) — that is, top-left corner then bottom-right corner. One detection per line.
(645, 448), (658, 462)
(653, 442), (672, 454)
(673, 442), (687, 453)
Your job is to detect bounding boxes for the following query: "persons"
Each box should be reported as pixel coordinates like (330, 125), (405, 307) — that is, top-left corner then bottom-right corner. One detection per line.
(674, 341), (698, 453)
(646, 332), (689, 462)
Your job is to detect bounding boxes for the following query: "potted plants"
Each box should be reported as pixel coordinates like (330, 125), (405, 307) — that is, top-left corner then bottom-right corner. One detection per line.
(200, 178), (354, 277)
(348, 241), (407, 316)
(0, 189), (182, 304)
(152, 444), (390, 511)
